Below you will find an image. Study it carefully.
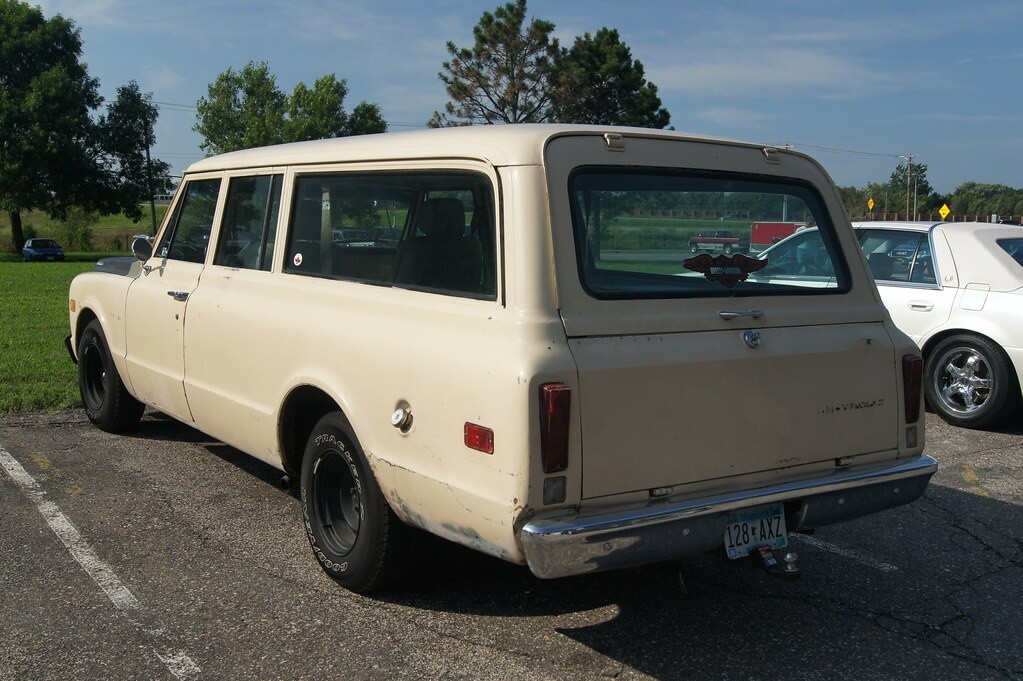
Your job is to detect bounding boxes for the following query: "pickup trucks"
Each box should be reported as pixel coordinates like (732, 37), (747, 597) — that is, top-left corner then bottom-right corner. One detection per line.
(687, 231), (750, 254)
(676, 220), (1023, 430)
(65, 123), (939, 597)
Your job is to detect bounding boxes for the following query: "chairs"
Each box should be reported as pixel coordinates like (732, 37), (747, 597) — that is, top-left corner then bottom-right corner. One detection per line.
(868, 253), (895, 280)
(243, 201), (341, 276)
(392, 197), (484, 294)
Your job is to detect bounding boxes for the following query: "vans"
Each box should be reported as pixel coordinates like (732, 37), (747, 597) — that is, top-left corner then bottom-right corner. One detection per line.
(154, 195), (173, 205)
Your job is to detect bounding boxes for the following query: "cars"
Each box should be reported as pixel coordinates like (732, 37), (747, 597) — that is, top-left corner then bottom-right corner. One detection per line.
(22, 238), (65, 262)
(997, 220), (1022, 226)
